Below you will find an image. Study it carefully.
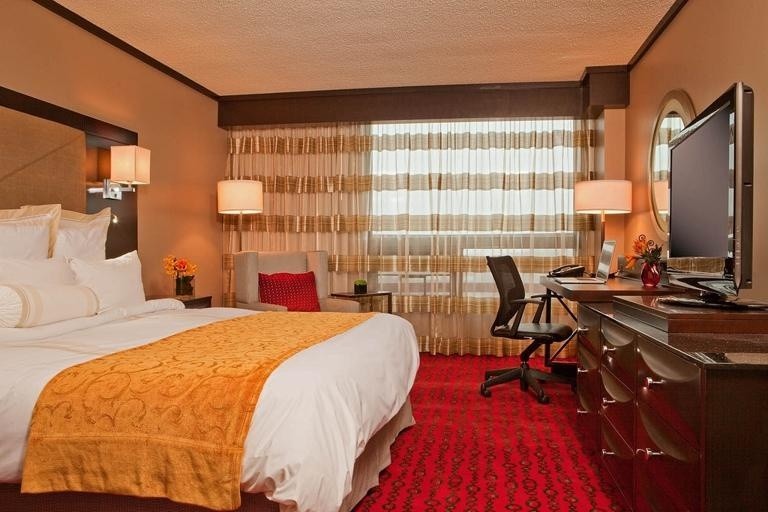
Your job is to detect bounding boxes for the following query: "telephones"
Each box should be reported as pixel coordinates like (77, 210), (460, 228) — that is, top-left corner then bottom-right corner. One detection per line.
(552, 264), (585, 276)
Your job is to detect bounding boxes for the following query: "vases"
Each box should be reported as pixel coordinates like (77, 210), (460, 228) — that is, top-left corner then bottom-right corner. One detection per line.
(641, 262), (660, 288)
(175, 277), (194, 296)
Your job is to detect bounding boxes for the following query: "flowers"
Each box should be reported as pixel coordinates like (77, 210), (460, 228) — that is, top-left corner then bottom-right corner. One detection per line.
(622, 234), (663, 272)
(158, 255), (199, 286)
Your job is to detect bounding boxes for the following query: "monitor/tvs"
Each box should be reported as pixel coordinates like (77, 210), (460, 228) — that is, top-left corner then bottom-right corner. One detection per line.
(657, 80), (768, 309)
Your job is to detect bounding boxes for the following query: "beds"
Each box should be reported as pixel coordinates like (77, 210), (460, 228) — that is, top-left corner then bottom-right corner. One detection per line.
(0, 85), (423, 511)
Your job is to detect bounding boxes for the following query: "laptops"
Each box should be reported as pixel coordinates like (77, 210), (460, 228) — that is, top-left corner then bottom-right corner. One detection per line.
(556, 240), (617, 284)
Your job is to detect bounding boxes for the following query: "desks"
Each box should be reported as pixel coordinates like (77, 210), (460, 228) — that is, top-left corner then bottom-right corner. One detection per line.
(539, 272), (686, 393)
(329, 291), (393, 314)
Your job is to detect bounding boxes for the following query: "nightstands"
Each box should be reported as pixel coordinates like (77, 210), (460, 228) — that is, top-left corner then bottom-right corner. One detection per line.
(149, 295), (214, 308)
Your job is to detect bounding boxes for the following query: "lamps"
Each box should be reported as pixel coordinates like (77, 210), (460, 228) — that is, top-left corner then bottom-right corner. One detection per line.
(217, 179), (265, 252)
(89, 144), (152, 201)
(573, 180), (633, 278)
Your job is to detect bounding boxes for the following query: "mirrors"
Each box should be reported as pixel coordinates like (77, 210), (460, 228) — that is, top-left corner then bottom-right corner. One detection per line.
(646, 89), (698, 242)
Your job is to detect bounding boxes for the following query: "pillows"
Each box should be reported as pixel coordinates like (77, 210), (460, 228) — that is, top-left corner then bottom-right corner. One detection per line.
(20, 204), (112, 263)
(0, 256), (80, 290)
(1, 203), (63, 261)
(0, 285), (100, 330)
(69, 250), (146, 323)
(256, 269), (322, 312)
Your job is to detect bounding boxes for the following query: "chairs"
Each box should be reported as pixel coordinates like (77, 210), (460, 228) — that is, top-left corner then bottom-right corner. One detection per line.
(480, 255), (574, 404)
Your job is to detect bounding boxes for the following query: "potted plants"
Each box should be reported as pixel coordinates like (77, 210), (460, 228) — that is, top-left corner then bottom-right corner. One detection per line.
(352, 279), (368, 293)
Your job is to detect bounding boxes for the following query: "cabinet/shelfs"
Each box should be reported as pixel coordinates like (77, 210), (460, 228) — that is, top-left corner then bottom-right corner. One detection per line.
(575, 302), (768, 511)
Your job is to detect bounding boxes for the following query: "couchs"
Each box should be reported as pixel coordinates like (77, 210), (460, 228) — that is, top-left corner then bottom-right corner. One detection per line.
(229, 249), (362, 312)
